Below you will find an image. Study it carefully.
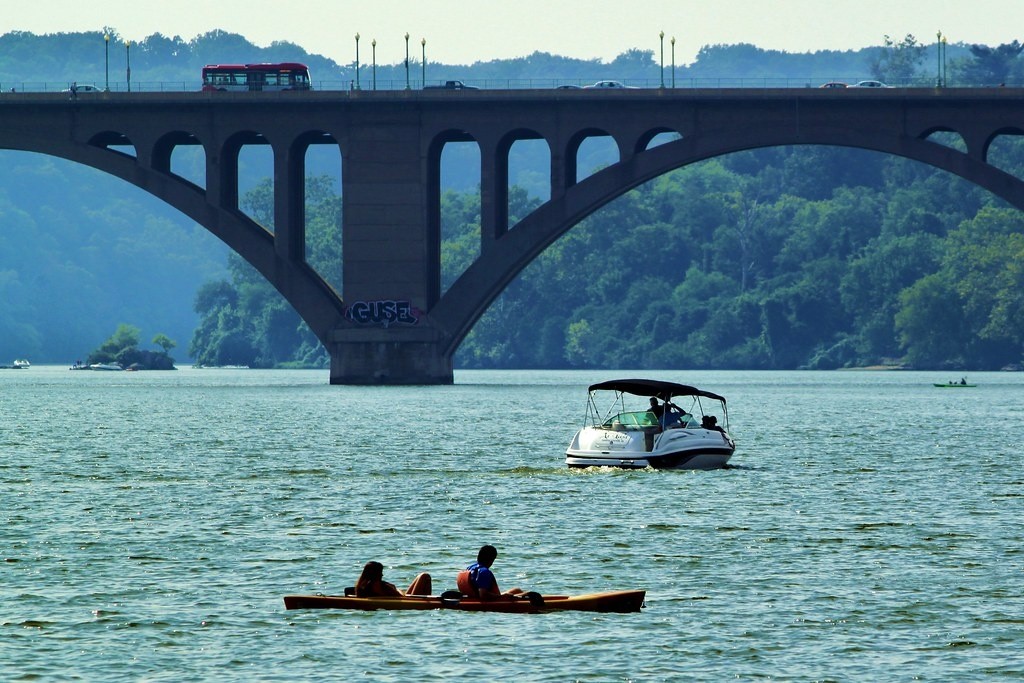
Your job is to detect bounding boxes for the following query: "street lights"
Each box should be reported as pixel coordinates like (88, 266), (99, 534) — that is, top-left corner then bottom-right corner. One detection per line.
(670, 36), (675, 89)
(371, 37), (377, 91)
(421, 38), (427, 86)
(935, 29), (948, 89)
(405, 32), (411, 91)
(103, 31), (112, 94)
(354, 31), (361, 91)
(125, 38), (132, 91)
(659, 29), (666, 89)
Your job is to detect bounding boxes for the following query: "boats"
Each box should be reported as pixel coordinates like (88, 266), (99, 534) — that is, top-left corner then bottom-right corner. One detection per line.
(286, 591), (647, 611)
(565, 378), (737, 470)
(931, 382), (979, 387)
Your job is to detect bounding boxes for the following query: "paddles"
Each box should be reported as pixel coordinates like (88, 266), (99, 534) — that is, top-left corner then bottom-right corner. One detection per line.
(458, 591), (546, 609)
(344, 586), (462, 609)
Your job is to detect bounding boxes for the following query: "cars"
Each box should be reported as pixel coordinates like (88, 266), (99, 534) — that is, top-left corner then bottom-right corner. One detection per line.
(554, 85), (584, 90)
(63, 86), (100, 92)
(846, 81), (896, 89)
(584, 81), (641, 90)
(819, 82), (848, 89)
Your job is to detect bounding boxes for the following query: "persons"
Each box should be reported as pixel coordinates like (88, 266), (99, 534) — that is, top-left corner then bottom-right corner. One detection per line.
(609, 82), (615, 86)
(869, 82), (875, 86)
(350, 80), (354, 89)
(467, 545), (522, 601)
(700, 416), (724, 432)
(659, 403), (686, 431)
(961, 378), (966, 385)
(646, 397), (663, 427)
(297, 78), (301, 82)
(218, 78), (229, 85)
(69, 82), (79, 101)
(356, 561), (432, 599)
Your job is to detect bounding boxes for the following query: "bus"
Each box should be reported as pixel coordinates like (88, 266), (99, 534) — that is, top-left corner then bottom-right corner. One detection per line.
(201, 62), (312, 91)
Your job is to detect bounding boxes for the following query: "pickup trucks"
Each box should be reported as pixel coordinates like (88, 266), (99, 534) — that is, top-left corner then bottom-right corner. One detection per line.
(424, 81), (479, 92)
